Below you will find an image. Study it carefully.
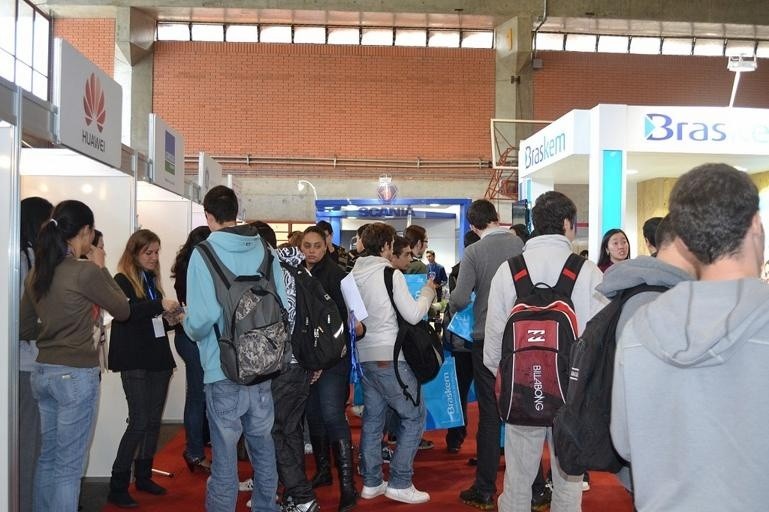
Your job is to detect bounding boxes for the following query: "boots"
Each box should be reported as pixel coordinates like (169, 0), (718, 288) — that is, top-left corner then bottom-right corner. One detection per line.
(307, 434), (333, 488)
(332, 437), (360, 511)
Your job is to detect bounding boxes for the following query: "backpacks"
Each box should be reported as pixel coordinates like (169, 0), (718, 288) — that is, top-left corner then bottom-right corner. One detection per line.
(382, 266), (446, 407)
(552, 304), (625, 474)
(494, 252), (579, 426)
(278, 259), (348, 372)
(191, 240), (293, 385)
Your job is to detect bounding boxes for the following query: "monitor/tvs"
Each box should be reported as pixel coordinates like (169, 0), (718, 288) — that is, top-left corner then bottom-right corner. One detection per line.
(512, 199), (530, 228)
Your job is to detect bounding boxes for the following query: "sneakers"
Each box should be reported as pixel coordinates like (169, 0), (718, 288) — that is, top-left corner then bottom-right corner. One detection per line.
(417, 438), (434, 450)
(107, 488), (140, 509)
(361, 480), (389, 499)
(446, 437), (463, 452)
(385, 486), (431, 504)
(237, 473), (321, 511)
(530, 467), (591, 510)
(470, 447), (504, 465)
(387, 431), (398, 445)
(459, 484), (496, 509)
(357, 446), (395, 464)
(134, 478), (167, 495)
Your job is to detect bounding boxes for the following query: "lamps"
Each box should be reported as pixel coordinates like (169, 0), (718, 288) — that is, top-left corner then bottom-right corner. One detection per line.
(727, 53), (758, 107)
(378, 177), (398, 202)
(297, 180), (317, 201)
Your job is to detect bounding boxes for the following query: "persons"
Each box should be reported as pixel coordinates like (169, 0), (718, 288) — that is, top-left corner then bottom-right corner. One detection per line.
(610, 161), (767, 511)
(23, 162), (705, 510)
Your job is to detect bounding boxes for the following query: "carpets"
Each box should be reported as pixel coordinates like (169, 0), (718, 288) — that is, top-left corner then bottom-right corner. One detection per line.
(98, 383), (635, 512)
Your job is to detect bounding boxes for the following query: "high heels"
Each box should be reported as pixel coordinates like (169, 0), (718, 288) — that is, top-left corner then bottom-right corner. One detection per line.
(182, 449), (212, 475)
